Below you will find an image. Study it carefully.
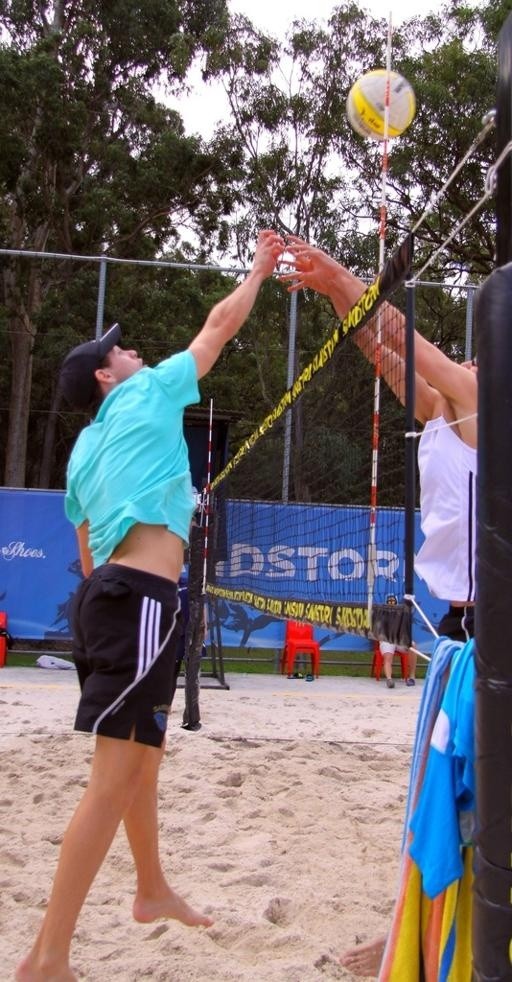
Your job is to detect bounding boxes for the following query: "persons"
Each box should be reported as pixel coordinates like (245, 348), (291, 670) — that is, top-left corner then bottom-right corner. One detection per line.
(376, 592), (417, 689)
(10, 221), (289, 982)
(272, 233), (478, 982)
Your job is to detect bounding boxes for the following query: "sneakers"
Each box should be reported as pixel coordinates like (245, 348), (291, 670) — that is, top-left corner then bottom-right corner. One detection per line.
(387, 678), (395, 688)
(406, 679), (415, 686)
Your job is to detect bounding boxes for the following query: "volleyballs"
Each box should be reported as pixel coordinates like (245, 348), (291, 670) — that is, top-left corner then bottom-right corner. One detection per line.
(345, 69), (416, 140)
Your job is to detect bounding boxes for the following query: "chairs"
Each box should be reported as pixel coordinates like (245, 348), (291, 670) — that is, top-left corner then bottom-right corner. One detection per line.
(371, 640), (412, 682)
(280, 618), (321, 679)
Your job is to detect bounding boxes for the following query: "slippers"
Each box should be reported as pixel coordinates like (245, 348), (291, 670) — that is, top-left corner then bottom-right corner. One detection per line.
(287, 672), (314, 682)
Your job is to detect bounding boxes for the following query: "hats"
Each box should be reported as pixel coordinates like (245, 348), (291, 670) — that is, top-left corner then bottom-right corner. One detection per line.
(60, 321), (124, 417)
